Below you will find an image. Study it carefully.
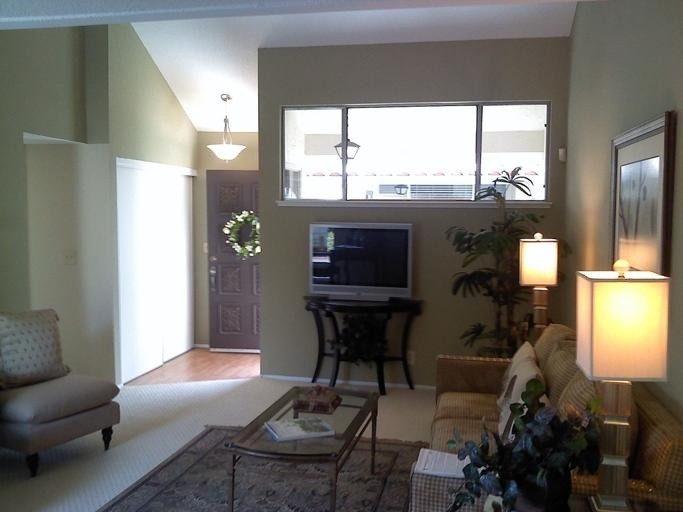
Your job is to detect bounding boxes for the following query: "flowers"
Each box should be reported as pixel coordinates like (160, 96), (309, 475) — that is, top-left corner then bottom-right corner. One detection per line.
(222, 207), (262, 261)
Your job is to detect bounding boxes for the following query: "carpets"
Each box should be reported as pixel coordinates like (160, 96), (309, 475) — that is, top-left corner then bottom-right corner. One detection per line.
(95, 421), (430, 512)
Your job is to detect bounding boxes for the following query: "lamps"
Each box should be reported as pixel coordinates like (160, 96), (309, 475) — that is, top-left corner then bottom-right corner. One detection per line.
(206, 92), (247, 164)
(517, 230), (562, 340)
(335, 138), (360, 161)
(571, 258), (675, 512)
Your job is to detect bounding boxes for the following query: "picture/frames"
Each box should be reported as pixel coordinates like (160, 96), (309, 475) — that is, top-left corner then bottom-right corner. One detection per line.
(604, 108), (678, 280)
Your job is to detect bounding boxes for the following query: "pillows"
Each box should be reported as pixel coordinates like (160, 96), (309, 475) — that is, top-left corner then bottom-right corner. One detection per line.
(494, 342), (549, 445)
(0, 309), (72, 391)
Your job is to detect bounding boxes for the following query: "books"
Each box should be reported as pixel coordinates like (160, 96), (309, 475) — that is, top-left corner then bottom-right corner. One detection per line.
(261, 414), (336, 442)
(413, 448), (472, 479)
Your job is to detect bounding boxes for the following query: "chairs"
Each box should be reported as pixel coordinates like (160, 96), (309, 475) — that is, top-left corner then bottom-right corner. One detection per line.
(0, 307), (124, 480)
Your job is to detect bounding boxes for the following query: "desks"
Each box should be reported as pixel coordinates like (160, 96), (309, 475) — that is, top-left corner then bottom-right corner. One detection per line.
(303, 295), (424, 397)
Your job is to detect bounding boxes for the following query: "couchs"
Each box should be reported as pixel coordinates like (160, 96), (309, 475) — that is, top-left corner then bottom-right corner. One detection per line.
(402, 320), (681, 510)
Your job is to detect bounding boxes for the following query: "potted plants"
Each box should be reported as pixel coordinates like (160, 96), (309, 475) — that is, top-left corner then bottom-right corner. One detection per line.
(441, 379), (605, 512)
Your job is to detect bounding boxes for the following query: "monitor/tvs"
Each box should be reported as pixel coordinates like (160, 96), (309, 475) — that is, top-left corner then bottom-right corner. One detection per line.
(308, 222), (414, 304)
